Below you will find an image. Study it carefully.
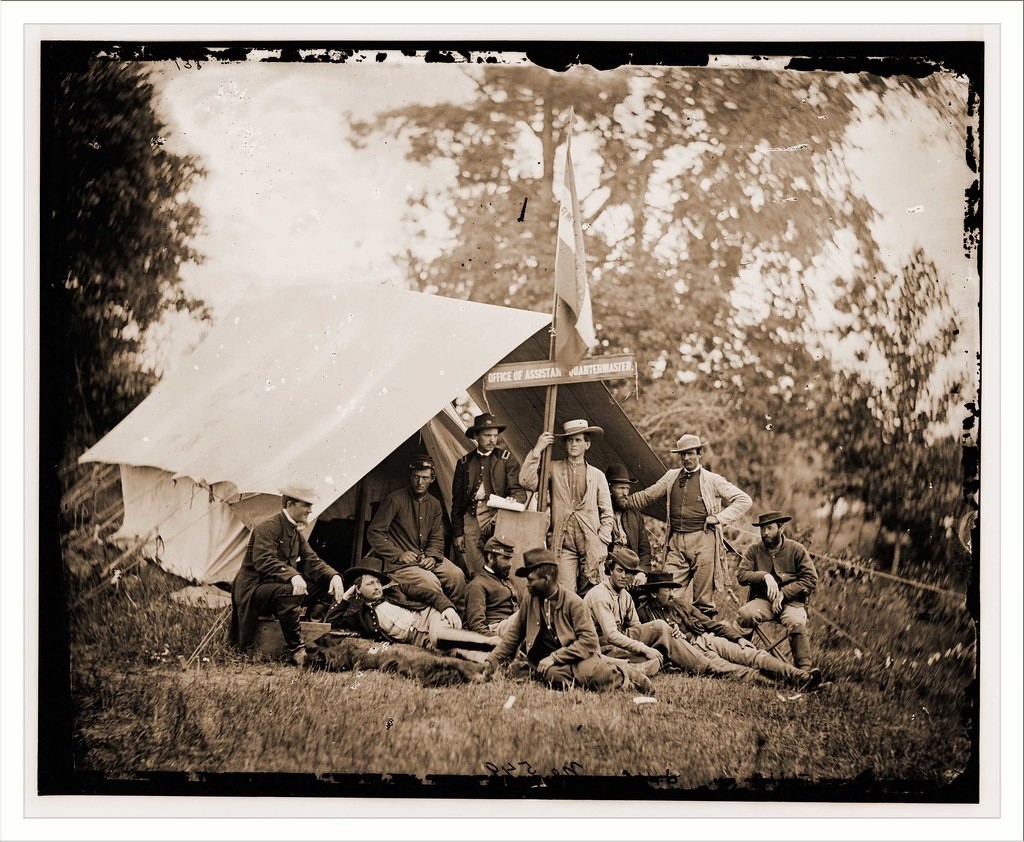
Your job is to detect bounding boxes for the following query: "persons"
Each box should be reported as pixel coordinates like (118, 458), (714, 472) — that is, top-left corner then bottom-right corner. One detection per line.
(627, 434), (754, 621)
(367, 454), (467, 628)
(581, 548), (665, 676)
(463, 534), (659, 679)
(518, 420), (614, 600)
(451, 413), (528, 580)
(638, 571), (822, 691)
(605, 464), (652, 607)
(232, 480), (343, 666)
(479, 547), (660, 698)
(733, 511), (822, 675)
(322, 556), (503, 664)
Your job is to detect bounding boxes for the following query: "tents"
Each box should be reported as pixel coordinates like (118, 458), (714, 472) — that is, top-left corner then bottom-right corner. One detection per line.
(80, 279), (736, 600)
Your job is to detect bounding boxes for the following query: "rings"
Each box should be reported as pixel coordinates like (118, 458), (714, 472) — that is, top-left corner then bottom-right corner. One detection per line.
(432, 563), (436, 567)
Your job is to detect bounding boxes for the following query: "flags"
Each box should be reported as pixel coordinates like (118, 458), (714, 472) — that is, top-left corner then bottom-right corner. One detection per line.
(546, 135), (596, 380)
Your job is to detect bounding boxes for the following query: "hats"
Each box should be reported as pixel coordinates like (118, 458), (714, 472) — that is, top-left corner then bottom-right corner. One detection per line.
(608, 547), (644, 573)
(344, 558), (392, 587)
(515, 547), (559, 577)
(603, 465), (641, 486)
(484, 534), (516, 557)
(465, 413), (507, 438)
(409, 454), (435, 470)
(638, 569), (683, 589)
(553, 419), (604, 442)
(752, 511), (792, 526)
(279, 481), (318, 505)
(669, 434), (709, 453)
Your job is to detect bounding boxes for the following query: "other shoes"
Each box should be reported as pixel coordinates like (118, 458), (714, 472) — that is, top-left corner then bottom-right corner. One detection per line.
(819, 681), (832, 692)
(627, 667), (655, 693)
(798, 667), (821, 688)
(290, 650), (309, 665)
